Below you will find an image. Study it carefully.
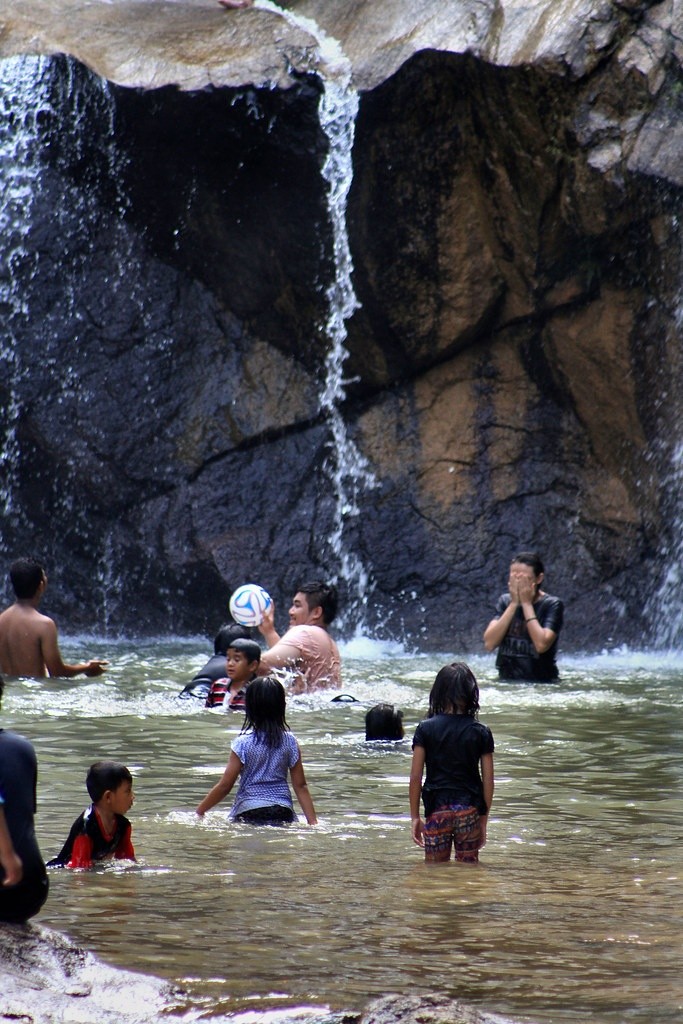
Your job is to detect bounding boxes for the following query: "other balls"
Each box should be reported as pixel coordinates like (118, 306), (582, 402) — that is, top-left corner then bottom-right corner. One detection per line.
(228, 582), (273, 628)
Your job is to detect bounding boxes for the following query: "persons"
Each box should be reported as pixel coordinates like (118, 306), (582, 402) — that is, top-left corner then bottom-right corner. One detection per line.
(0, 678), (49, 926)
(0, 557), (110, 678)
(409, 663), (494, 861)
(46, 760), (136, 866)
(365, 704), (404, 740)
(179, 623), (251, 698)
(206, 638), (261, 709)
(484, 554), (565, 684)
(196, 677), (317, 823)
(255, 580), (341, 695)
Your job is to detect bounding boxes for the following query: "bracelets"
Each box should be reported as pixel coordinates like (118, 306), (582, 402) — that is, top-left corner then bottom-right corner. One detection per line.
(526, 618), (538, 623)
